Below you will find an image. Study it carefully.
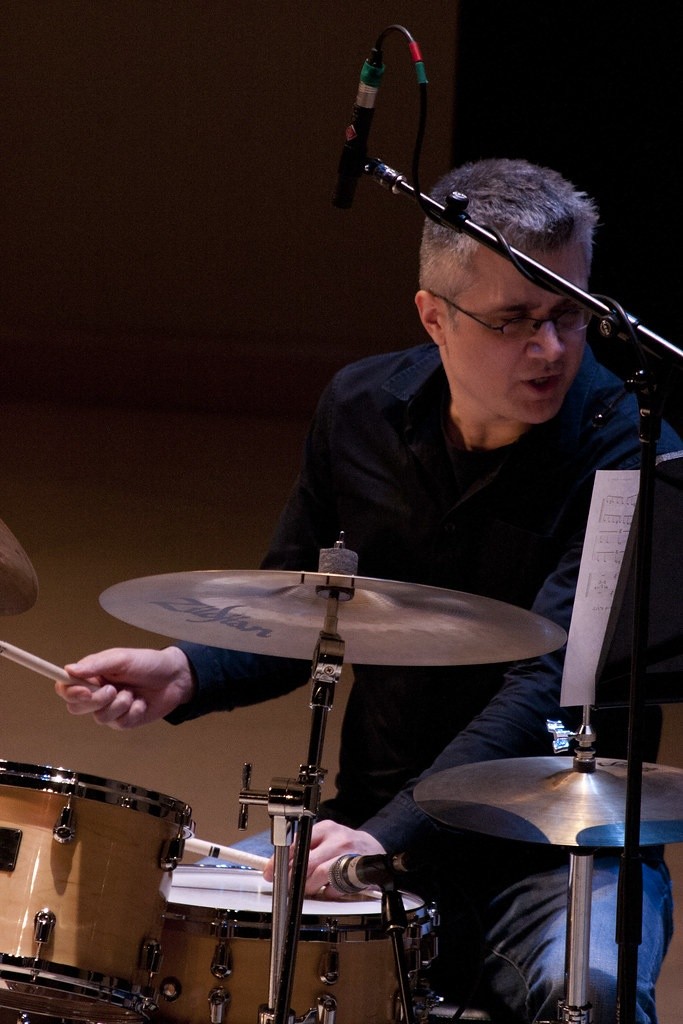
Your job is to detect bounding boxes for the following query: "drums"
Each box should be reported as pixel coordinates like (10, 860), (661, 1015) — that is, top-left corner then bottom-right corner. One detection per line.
(156, 864), (441, 1024)
(0, 758), (194, 1024)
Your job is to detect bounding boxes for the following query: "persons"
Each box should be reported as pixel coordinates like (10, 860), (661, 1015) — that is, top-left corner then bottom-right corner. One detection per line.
(57, 159), (683, 1024)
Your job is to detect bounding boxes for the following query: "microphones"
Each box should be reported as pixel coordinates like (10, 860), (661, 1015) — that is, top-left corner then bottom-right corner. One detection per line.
(327, 852), (415, 894)
(330, 47), (388, 210)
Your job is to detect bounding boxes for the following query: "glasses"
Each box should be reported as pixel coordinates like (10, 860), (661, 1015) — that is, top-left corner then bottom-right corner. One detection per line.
(426, 288), (593, 342)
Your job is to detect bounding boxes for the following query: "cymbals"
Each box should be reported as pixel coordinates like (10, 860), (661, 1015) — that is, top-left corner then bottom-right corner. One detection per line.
(412, 755), (683, 848)
(100, 569), (567, 667)
(0, 517), (40, 619)
